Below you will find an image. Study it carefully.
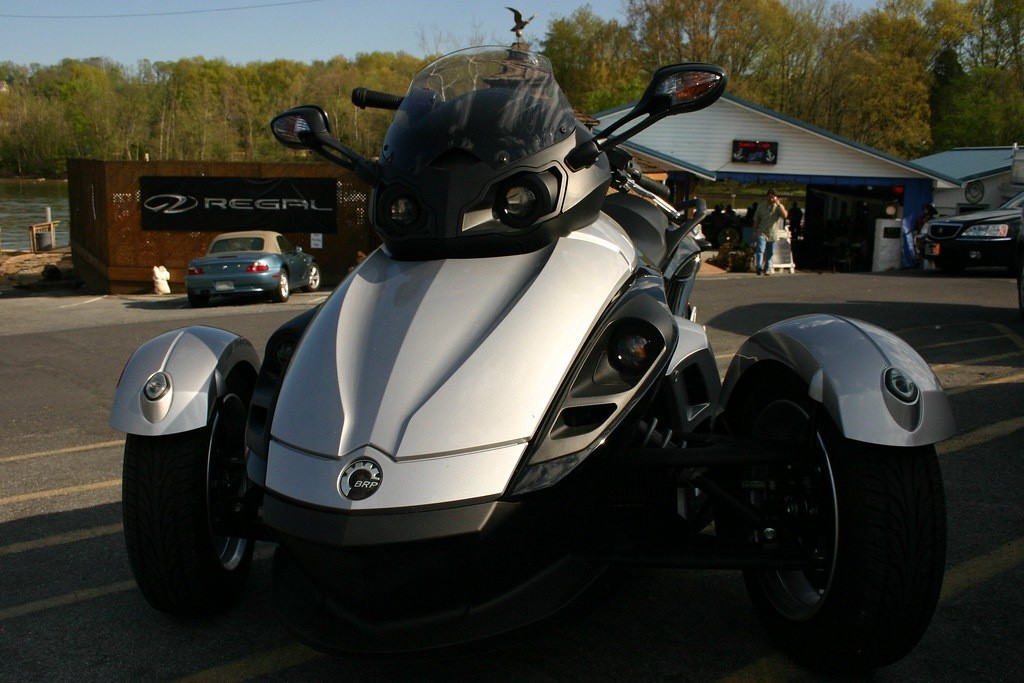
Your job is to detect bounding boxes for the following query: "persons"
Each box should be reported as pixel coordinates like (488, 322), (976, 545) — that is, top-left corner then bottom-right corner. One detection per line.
(788, 202), (802, 240)
(751, 189), (788, 275)
(711, 202), (758, 249)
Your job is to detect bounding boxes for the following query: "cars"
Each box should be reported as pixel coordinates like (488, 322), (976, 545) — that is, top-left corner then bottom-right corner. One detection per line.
(912, 188), (1024, 276)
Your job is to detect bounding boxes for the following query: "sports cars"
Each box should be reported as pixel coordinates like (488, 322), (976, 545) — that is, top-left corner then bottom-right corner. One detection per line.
(184, 231), (321, 308)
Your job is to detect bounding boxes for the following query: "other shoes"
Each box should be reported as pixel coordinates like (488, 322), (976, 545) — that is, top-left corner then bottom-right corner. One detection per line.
(757, 268), (762, 275)
(765, 271), (770, 275)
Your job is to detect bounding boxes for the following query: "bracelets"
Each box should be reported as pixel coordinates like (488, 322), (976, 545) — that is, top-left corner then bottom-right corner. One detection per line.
(753, 229), (757, 232)
(778, 205), (780, 207)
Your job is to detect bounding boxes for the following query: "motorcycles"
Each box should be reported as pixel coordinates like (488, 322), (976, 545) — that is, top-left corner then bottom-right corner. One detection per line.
(99, 39), (957, 677)
